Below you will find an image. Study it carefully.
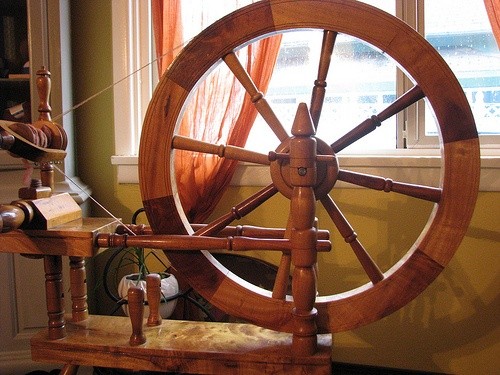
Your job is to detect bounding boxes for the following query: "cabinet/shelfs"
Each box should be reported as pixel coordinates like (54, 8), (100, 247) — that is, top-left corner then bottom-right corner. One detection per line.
(1, 0), (78, 375)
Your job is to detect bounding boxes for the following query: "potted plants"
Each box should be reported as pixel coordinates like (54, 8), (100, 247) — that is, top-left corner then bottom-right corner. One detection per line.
(86, 246), (179, 320)
